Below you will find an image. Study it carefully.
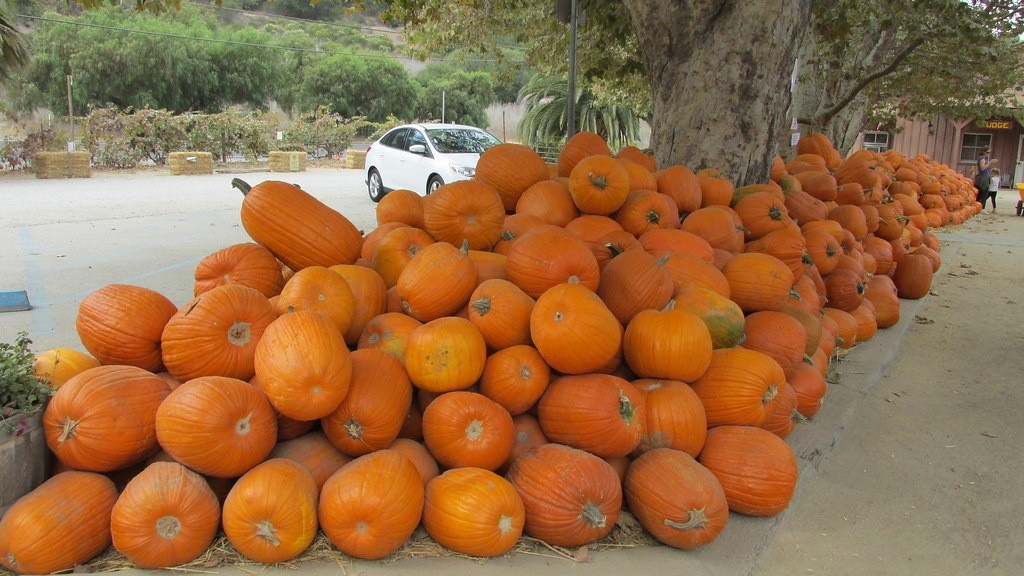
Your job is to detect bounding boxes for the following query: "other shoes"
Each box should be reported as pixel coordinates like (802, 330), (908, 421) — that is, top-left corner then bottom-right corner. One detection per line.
(982, 209), (990, 215)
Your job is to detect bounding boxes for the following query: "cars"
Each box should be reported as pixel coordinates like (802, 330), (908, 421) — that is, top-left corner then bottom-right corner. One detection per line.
(365, 123), (503, 201)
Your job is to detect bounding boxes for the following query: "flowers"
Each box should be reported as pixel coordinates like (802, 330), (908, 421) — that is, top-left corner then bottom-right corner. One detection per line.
(1, 330), (63, 437)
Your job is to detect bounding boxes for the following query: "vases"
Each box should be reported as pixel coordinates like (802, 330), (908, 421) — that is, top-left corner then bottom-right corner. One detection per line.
(0, 401), (44, 446)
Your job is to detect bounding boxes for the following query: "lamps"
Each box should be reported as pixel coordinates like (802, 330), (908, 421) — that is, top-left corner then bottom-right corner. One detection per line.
(926, 122), (933, 134)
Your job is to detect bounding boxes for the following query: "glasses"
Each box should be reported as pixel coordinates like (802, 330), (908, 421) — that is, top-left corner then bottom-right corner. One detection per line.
(986, 151), (990, 154)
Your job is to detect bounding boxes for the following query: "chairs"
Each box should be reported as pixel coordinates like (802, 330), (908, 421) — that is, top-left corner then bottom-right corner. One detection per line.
(999, 173), (1013, 189)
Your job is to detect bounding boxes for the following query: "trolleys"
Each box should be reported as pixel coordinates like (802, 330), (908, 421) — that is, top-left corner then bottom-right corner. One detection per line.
(1015, 181), (1024, 217)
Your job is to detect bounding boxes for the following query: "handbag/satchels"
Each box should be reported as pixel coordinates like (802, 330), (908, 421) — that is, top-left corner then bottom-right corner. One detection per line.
(973, 171), (990, 191)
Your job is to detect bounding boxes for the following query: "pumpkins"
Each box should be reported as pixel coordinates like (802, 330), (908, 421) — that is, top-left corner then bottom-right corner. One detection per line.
(0, 131), (983, 575)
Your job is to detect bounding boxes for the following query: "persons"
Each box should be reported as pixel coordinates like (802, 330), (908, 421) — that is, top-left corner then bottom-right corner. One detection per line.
(983, 168), (1001, 211)
(976, 144), (999, 213)
